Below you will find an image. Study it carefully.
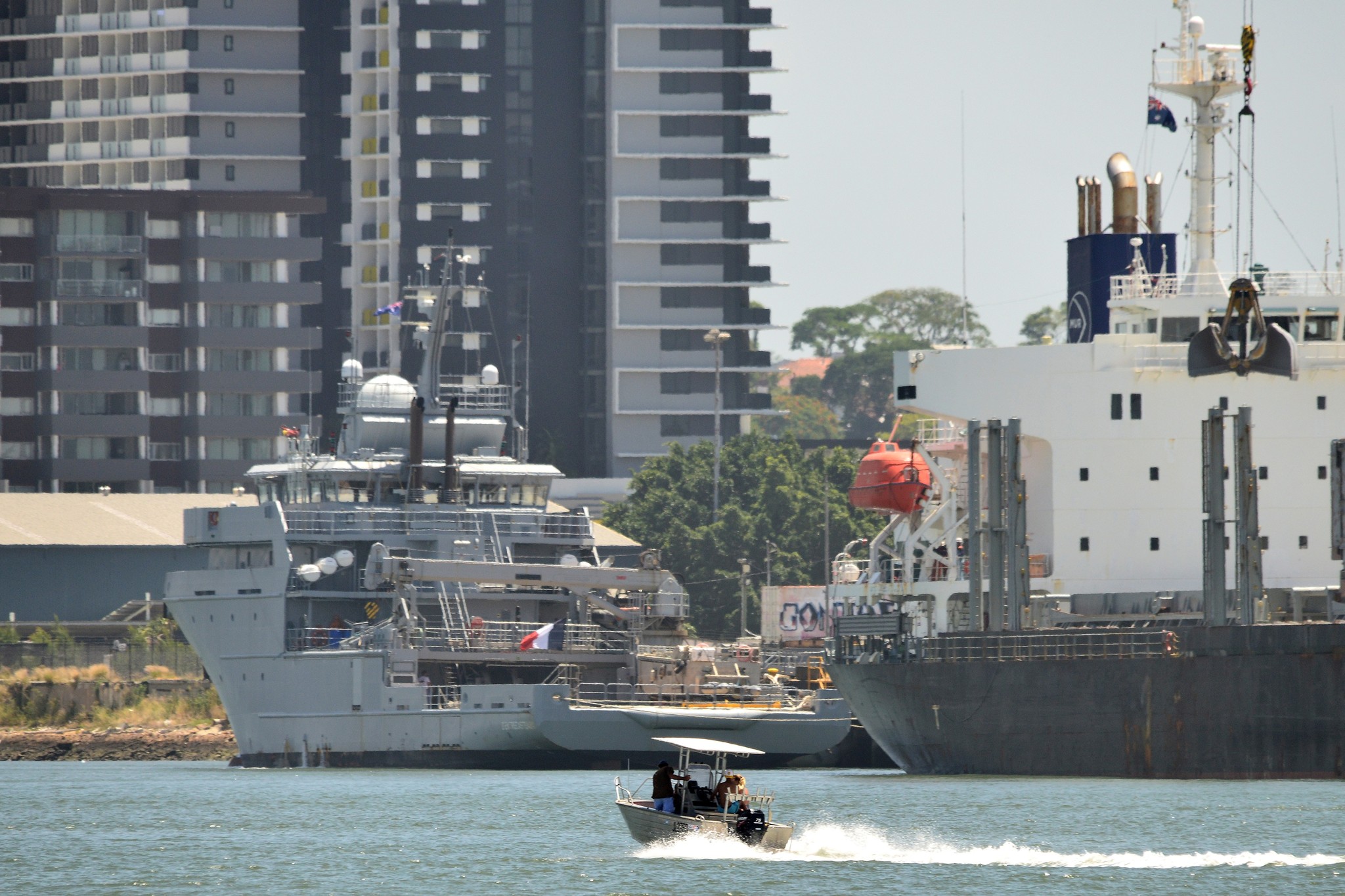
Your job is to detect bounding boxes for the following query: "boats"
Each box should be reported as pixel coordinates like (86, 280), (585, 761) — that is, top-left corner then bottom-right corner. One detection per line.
(161, 230), (853, 770)
(613, 736), (796, 852)
(847, 440), (934, 515)
(821, 0), (1345, 781)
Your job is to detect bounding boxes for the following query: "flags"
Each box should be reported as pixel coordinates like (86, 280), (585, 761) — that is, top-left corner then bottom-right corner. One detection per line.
(520, 617), (565, 651)
(1148, 96), (1177, 133)
(373, 300), (403, 317)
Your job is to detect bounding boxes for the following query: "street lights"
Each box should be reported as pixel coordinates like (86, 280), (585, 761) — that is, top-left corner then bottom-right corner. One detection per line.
(704, 329), (732, 523)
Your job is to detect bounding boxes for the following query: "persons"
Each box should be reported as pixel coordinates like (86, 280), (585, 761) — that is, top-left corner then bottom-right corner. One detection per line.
(732, 774), (748, 804)
(419, 670), (431, 709)
(710, 768), (748, 814)
(651, 761), (691, 813)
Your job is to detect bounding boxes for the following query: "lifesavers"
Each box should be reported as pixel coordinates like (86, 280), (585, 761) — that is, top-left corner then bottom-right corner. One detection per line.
(736, 644), (752, 662)
(312, 630), (328, 646)
(964, 560), (970, 575)
(695, 643), (709, 647)
(209, 512), (219, 525)
